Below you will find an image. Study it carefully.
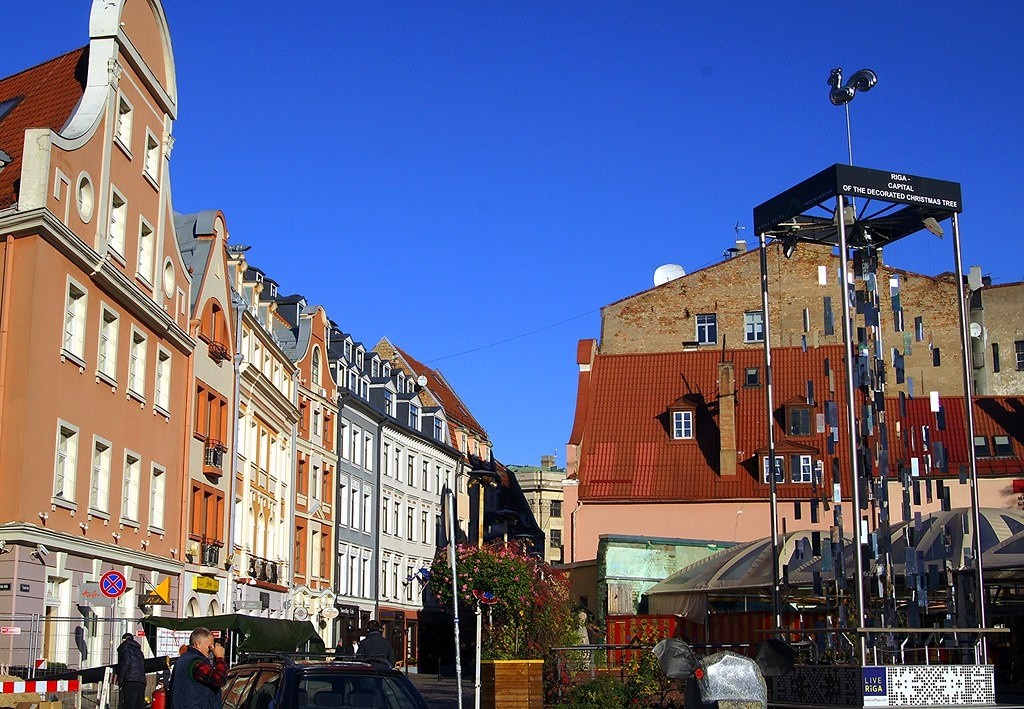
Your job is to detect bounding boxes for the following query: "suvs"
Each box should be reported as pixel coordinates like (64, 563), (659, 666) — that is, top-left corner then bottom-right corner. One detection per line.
(220, 652), (431, 709)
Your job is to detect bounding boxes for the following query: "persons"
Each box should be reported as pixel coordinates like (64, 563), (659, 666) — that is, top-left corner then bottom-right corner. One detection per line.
(348, 635), (360, 658)
(353, 619), (396, 690)
(116, 633), (148, 709)
(333, 639), (348, 660)
(166, 626), (227, 708)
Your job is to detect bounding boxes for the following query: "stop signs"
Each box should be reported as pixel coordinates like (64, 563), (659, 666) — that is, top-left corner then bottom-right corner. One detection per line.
(100, 570), (126, 599)
